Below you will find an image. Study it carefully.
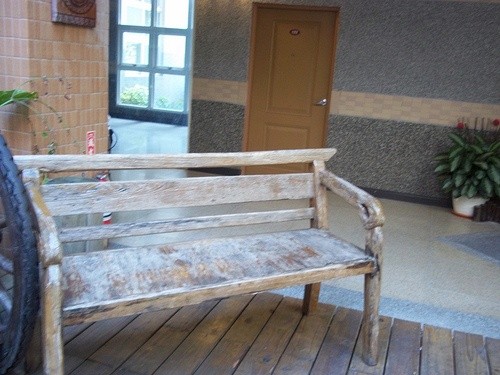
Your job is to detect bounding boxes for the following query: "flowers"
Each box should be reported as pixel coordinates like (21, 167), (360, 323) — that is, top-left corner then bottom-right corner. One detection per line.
(432, 115), (500, 199)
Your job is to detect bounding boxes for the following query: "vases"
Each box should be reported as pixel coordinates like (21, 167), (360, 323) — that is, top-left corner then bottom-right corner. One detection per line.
(453, 191), (489, 220)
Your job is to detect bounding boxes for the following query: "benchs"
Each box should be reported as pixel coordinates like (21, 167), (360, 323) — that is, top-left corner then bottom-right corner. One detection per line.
(11, 147), (386, 375)
